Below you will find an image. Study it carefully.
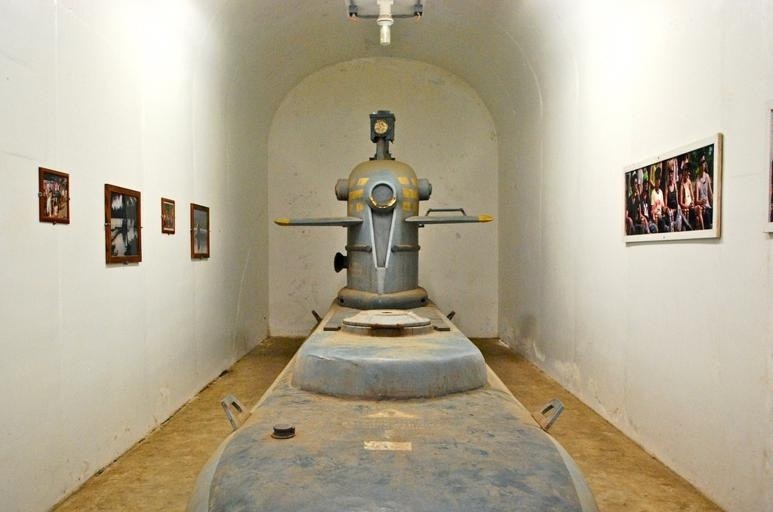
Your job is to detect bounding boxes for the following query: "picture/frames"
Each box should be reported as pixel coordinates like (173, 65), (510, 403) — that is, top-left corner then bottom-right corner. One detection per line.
(620, 132), (722, 242)
(37, 166), (69, 225)
(189, 202), (209, 259)
(160, 197), (175, 234)
(103, 183), (141, 264)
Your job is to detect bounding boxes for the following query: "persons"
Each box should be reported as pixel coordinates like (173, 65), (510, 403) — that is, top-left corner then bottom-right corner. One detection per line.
(45, 180), (61, 217)
(625, 154), (713, 235)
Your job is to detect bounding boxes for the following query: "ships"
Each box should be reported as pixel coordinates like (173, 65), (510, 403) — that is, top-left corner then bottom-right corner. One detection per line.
(183, 110), (598, 512)
